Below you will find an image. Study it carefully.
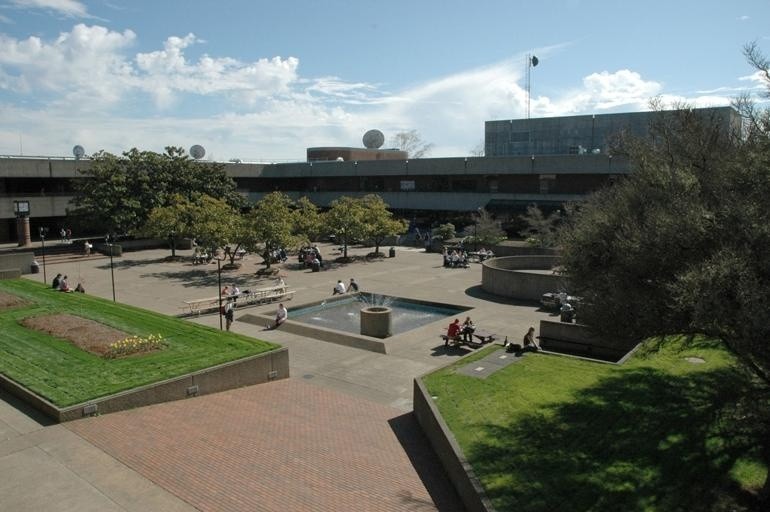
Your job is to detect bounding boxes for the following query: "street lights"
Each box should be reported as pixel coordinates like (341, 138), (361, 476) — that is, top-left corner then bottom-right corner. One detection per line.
(39, 227), (49, 284)
(471, 213), (480, 251)
(208, 240), (230, 331)
(105, 232), (119, 303)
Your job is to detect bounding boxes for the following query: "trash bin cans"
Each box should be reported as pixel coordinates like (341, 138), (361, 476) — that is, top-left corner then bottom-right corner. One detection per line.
(389, 246), (395, 257)
(30, 261), (39, 274)
(561, 305), (573, 323)
(312, 259), (320, 272)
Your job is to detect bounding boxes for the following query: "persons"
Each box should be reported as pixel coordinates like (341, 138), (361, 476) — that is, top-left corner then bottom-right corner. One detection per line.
(446, 319), (461, 350)
(230, 284), (239, 298)
(74, 283), (85, 293)
(223, 297), (234, 330)
(462, 316), (476, 343)
(524, 327), (543, 350)
(442, 249), (468, 267)
(191, 248), (211, 265)
(264, 303), (287, 331)
(332, 280), (346, 295)
(60, 276), (71, 290)
(222, 286), (232, 301)
(264, 243), (322, 267)
(58, 227), (98, 256)
(53, 273), (60, 288)
(486, 248), (494, 258)
(477, 248), (487, 262)
(273, 276), (284, 292)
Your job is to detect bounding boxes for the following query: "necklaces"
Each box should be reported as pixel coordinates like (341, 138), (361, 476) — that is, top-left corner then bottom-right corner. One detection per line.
(346, 278), (358, 292)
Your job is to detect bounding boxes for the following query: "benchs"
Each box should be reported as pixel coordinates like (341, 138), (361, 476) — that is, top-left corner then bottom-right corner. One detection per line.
(439, 320), (497, 353)
(177, 283), (297, 318)
